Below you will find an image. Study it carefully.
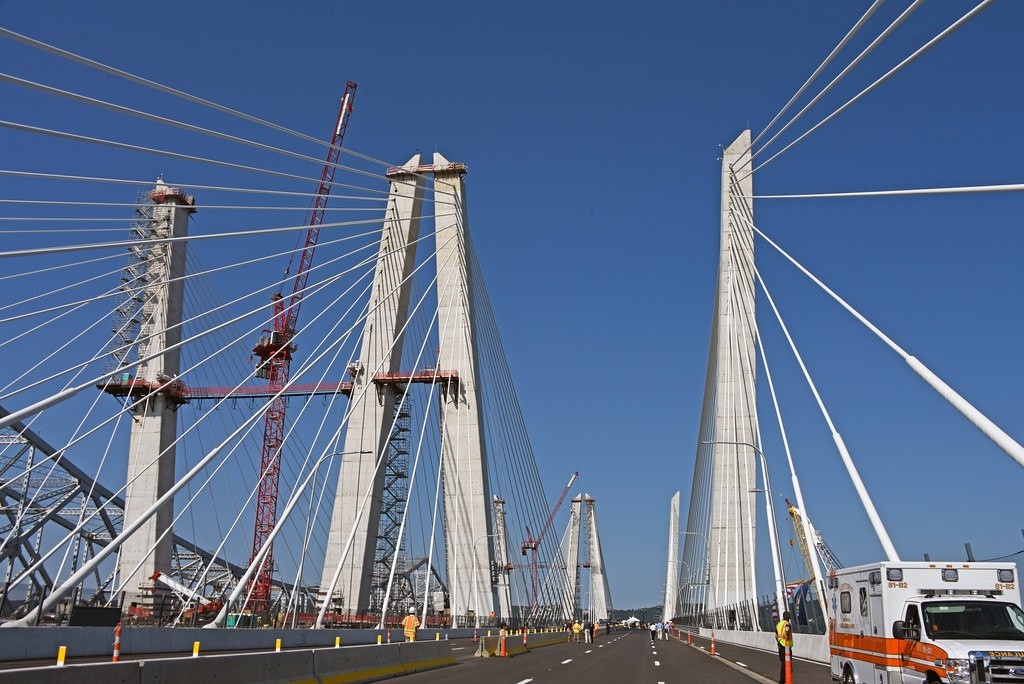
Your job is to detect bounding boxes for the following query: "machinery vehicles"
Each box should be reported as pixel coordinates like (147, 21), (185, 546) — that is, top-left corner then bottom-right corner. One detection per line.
(786, 498), (844, 621)
(149, 571), (224, 626)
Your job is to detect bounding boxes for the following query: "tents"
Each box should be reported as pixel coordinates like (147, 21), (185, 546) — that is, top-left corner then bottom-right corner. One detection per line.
(627, 616), (640, 622)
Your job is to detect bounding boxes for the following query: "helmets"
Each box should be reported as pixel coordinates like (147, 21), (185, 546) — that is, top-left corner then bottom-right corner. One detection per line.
(575, 620), (579, 622)
(409, 607), (416, 613)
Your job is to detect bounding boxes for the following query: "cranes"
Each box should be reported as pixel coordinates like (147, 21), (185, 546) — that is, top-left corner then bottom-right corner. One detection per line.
(506, 471), (580, 617)
(95, 80), (458, 627)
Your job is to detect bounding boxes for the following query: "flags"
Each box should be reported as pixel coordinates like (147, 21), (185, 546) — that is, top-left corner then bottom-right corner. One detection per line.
(792, 580), (813, 625)
(772, 582), (800, 620)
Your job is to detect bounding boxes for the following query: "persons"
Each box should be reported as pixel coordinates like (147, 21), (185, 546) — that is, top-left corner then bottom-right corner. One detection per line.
(657, 621), (663, 641)
(499, 618), (510, 635)
(630, 622), (650, 631)
(775, 610), (794, 684)
(582, 618), (593, 643)
(649, 623), (657, 640)
(401, 606), (421, 643)
(565, 620), (572, 642)
(665, 621), (670, 641)
(590, 623), (595, 643)
(606, 621), (610, 634)
(277, 609), (286, 628)
(572, 619), (582, 642)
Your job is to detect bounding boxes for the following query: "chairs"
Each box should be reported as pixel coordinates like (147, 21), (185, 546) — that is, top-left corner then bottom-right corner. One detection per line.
(972, 613), (994, 633)
(940, 613), (965, 631)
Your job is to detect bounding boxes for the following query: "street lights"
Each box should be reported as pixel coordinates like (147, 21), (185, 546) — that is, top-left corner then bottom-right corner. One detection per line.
(653, 531), (709, 635)
(700, 441), (790, 617)
(293, 451), (373, 628)
(465, 535), (591, 630)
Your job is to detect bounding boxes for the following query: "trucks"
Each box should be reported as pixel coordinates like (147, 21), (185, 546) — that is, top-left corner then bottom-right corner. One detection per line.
(828, 562), (1024, 684)
